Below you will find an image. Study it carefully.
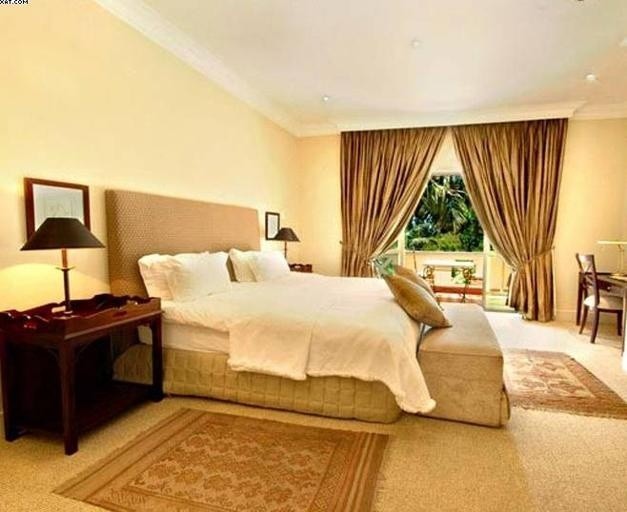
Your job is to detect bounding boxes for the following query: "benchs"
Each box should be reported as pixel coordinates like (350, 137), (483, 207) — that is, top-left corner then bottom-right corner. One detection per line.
(414, 300), (505, 431)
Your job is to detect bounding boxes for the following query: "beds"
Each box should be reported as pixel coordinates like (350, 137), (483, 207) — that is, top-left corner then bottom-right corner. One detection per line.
(101, 188), (420, 426)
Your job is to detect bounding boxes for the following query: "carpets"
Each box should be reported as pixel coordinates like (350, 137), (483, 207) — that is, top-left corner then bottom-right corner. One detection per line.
(49, 405), (397, 512)
(496, 346), (627, 421)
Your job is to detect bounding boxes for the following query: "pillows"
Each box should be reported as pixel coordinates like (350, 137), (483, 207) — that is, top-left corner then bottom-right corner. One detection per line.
(137, 249), (211, 303)
(379, 272), (453, 330)
(228, 246), (255, 283)
(393, 263), (444, 310)
(163, 249), (233, 299)
(244, 250), (292, 283)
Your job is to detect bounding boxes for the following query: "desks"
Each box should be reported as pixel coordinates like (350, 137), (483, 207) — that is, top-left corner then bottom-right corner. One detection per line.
(0, 294), (164, 457)
(419, 257), (476, 304)
(574, 270), (627, 336)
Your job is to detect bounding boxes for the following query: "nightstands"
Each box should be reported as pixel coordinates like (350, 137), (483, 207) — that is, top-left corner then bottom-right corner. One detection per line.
(288, 262), (314, 273)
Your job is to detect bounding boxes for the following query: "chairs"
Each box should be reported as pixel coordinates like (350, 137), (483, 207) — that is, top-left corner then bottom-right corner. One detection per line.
(574, 252), (623, 343)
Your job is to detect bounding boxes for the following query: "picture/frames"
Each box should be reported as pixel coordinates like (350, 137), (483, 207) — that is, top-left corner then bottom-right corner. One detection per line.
(23, 176), (92, 244)
(264, 211), (281, 241)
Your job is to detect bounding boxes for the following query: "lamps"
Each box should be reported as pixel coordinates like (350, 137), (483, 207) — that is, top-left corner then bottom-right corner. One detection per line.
(273, 227), (302, 260)
(17, 217), (107, 315)
(597, 240), (626, 280)
